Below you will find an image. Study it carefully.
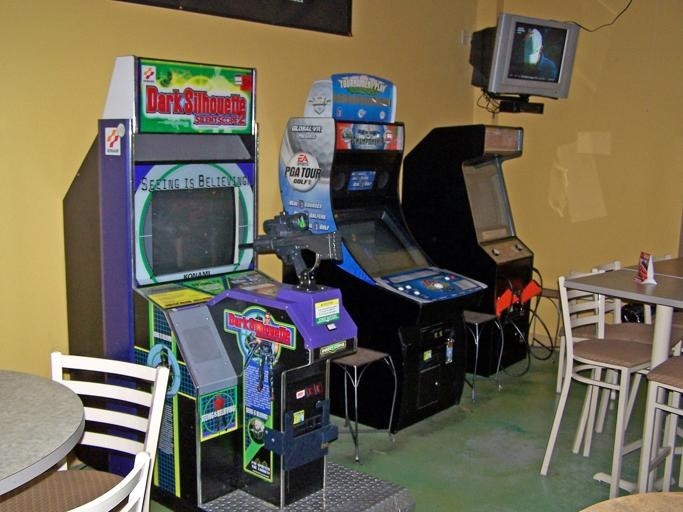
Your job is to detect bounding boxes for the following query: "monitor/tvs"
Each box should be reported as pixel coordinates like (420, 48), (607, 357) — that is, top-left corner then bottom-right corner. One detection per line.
(336, 218), (417, 276)
(468, 12), (581, 100)
(466, 171), (503, 230)
(151, 186), (236, 276)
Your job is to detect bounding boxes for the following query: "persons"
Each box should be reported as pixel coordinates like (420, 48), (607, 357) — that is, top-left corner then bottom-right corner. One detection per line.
(513, 29), (557, 80)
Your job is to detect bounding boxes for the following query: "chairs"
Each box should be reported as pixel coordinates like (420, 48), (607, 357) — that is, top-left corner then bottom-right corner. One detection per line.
(0, 350), (170, 512)
(540, 250), (683, 497)
